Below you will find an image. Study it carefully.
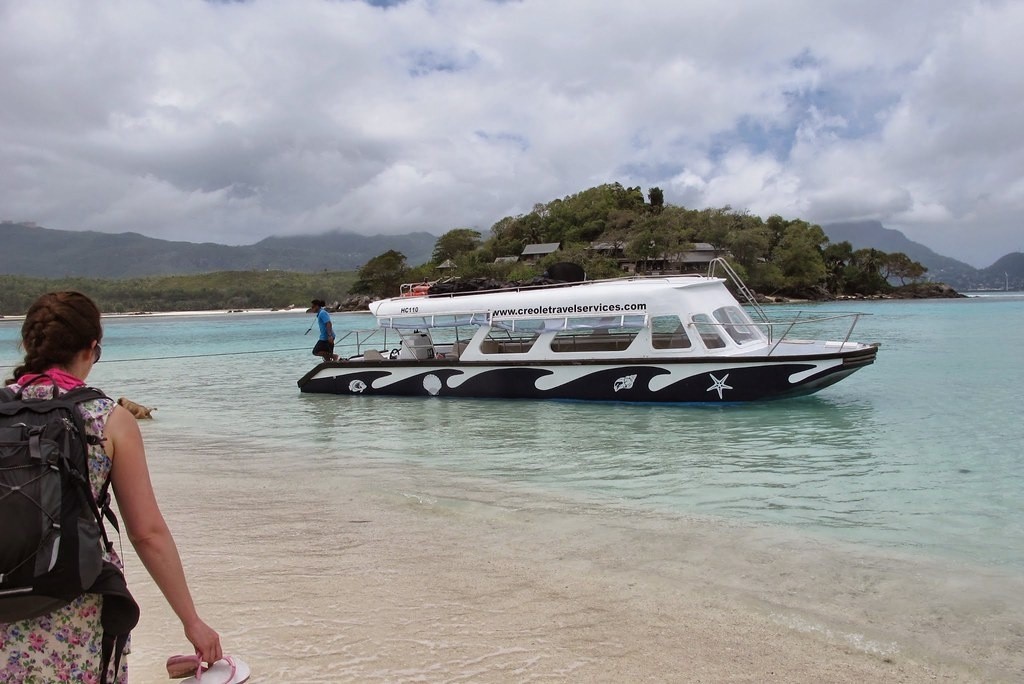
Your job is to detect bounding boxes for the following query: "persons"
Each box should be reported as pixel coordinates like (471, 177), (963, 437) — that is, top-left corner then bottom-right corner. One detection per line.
(0, 291), (223, 684)
(311, 300), (338, 361)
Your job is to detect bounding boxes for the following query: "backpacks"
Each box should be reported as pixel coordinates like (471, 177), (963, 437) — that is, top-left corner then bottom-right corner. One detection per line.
(0, 373), (114, 622)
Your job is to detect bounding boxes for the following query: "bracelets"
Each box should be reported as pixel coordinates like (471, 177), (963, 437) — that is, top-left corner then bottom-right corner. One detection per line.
(328, 335), (332, 337)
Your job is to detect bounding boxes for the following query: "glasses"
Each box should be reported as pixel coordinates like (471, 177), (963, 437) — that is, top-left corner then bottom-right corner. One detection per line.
(93, 345), (101, 364)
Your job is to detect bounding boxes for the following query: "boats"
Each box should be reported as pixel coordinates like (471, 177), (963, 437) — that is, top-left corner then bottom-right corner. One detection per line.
(295, 257), (882, 408)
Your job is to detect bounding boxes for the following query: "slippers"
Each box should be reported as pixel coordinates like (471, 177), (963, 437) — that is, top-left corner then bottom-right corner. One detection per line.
(180, 654), (250, 684)
(167, 653), (212, 679)
